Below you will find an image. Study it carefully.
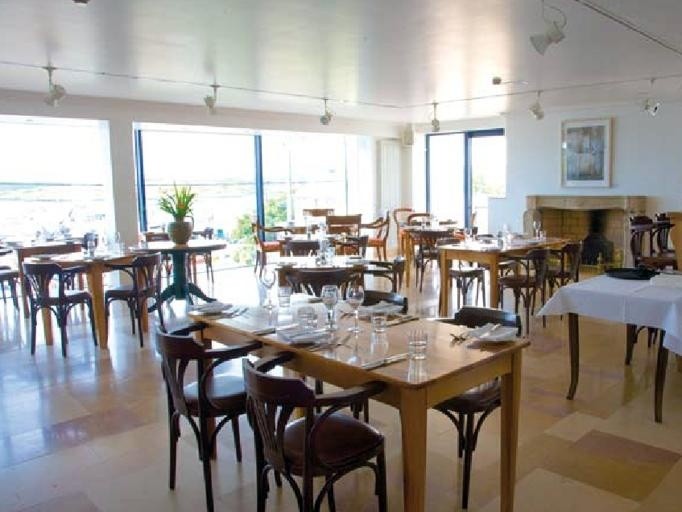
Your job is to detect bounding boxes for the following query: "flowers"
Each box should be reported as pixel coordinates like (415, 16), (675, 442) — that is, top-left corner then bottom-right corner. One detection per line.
(155, 180), (198, 223)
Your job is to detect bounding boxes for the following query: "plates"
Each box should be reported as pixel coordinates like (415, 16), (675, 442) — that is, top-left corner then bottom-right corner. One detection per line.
(193, 305), (231, 314)
(32, 254), (57, 260)
(471, 327), (517, 343)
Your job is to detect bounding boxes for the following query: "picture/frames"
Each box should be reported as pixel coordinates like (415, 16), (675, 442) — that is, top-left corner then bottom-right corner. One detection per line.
(558, 115), (615, 188)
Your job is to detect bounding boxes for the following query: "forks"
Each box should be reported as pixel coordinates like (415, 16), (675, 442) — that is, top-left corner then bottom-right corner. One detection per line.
(305, 332), (352, 352)
(339, 309), (352, 314)
(449, 330), (467, 341)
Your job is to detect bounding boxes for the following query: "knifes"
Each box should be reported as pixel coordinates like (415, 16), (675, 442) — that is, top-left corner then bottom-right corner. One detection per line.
(387, 314), (419, 327)
(362, 352), (407, 370)
(252, 324), (297, 337)
(227, 306), (249, 318)
(480, 323), (502, 338)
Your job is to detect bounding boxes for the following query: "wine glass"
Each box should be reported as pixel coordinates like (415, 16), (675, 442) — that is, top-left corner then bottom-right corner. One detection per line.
(347, 333), (362, 366)
(345, 285), (366, 334)
(260, 268), (276, 310)
(462, 218), (546, 250)
(322, 285), (339, 331)
(277, 287), (293, 307)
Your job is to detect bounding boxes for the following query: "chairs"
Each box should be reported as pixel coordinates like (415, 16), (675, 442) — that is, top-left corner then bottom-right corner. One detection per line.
(374, 307), (522, 510)
(317, 290), (409, 423)
(394, 208), (482, 292)
(629, 215), (671, 344)
(433, 234), (583, 335)
(252, 208), (389, 279)
(242, 349), (387, 511)
(654, 211), (682, 282)
(153, 323), (261, 511)
(1, 227), (213, 357)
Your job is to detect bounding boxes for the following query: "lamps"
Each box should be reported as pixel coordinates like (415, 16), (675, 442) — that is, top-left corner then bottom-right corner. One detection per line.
(201, 75), (682, 132)
(529, 0), (568, 56)
(39, 66), (68, 105)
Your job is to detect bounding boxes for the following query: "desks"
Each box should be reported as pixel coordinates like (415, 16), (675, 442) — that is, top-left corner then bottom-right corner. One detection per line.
(557, 273), (682, 423)
(5, 238), (87, 318)
(437, 236), (571, 318)
(127, 239), (226, 316)
(275, 254), (366, 299)
(23, 250), (150, 349)
(187, 292), (532, 512)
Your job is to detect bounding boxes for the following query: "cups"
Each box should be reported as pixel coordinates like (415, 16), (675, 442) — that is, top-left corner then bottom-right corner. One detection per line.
(300, 313), (318, 329)
(264, 308), (278, 327)
(421, 215), (438, 231)
(88, 240), (95, 253)
(369, 332), (390, 353)
(370, 305), (387, 331)
(408, 359), (426, 384)
(408, 330), (428, 361)
